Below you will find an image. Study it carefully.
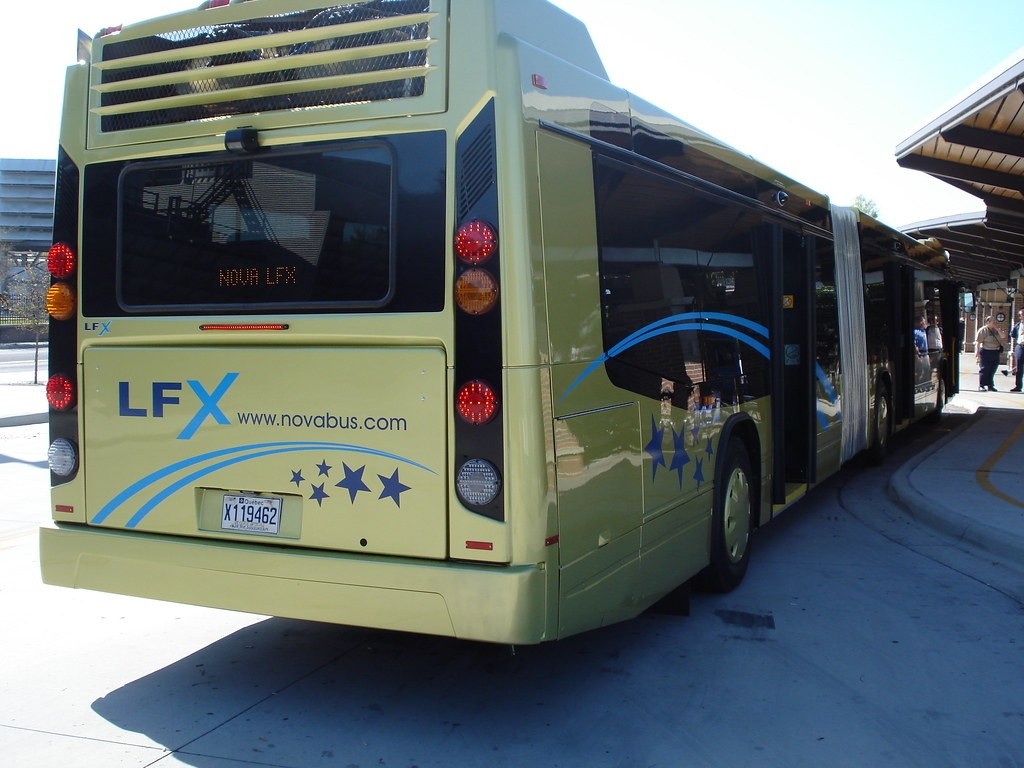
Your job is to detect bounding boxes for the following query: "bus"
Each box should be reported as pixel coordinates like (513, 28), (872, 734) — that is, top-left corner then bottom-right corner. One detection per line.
(39, 0), (976, 645)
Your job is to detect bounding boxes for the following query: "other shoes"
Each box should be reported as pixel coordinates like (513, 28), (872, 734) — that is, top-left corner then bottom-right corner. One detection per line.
(988, 387), (997, 391)
(979, 387), (986, 391)
(1010, 387), (1021, 391)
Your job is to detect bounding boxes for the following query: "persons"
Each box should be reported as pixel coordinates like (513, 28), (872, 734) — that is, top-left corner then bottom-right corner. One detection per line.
(1009, 309), (1024, 392)
(975, 316), (1003, 392)
(959, 317), (965, 353)
(914, 315), (943, 392)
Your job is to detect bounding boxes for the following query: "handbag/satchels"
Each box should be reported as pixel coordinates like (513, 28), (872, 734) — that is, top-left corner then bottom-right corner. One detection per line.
(999, 346), (1003, 353)
